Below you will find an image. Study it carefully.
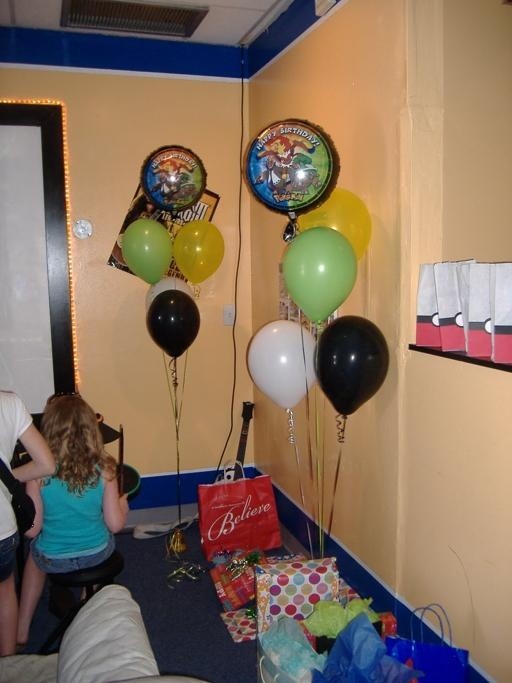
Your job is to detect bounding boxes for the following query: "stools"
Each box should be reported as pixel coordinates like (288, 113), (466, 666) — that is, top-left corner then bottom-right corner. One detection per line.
(37, 550), (124, 655)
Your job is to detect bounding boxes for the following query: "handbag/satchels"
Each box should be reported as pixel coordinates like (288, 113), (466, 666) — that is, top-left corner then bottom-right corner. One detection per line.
(254, 616), (325, 683)
(385, 604), (469, 683)
(12, 493), (36, 533)
(199, 459), (283, 561)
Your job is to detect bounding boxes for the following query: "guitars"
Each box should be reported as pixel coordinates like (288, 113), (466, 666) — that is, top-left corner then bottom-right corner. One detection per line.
(219, 402), (255, 480)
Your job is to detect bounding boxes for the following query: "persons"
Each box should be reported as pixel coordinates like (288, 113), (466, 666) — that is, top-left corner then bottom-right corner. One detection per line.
(15, 391), (129, 645)
(1, 389), (55, 656)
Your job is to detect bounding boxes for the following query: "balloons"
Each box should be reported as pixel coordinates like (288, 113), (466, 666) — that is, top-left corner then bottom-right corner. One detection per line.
(138, 144), (207, 211)
(313, 314), (389, 414)
(281, 226), (358, 324)
(144, 275), (194, 312)
(120, 218), (171, 284)
(145, 289), (200, 358)
(243, 117), (341, 217)
(245, 319), (316, 410)
(296, 186), (372, 261)
(172, 218), (226, 284)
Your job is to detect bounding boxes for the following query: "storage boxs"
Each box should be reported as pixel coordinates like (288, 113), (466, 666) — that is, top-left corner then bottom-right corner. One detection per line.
(210, 548), (359, 636)
